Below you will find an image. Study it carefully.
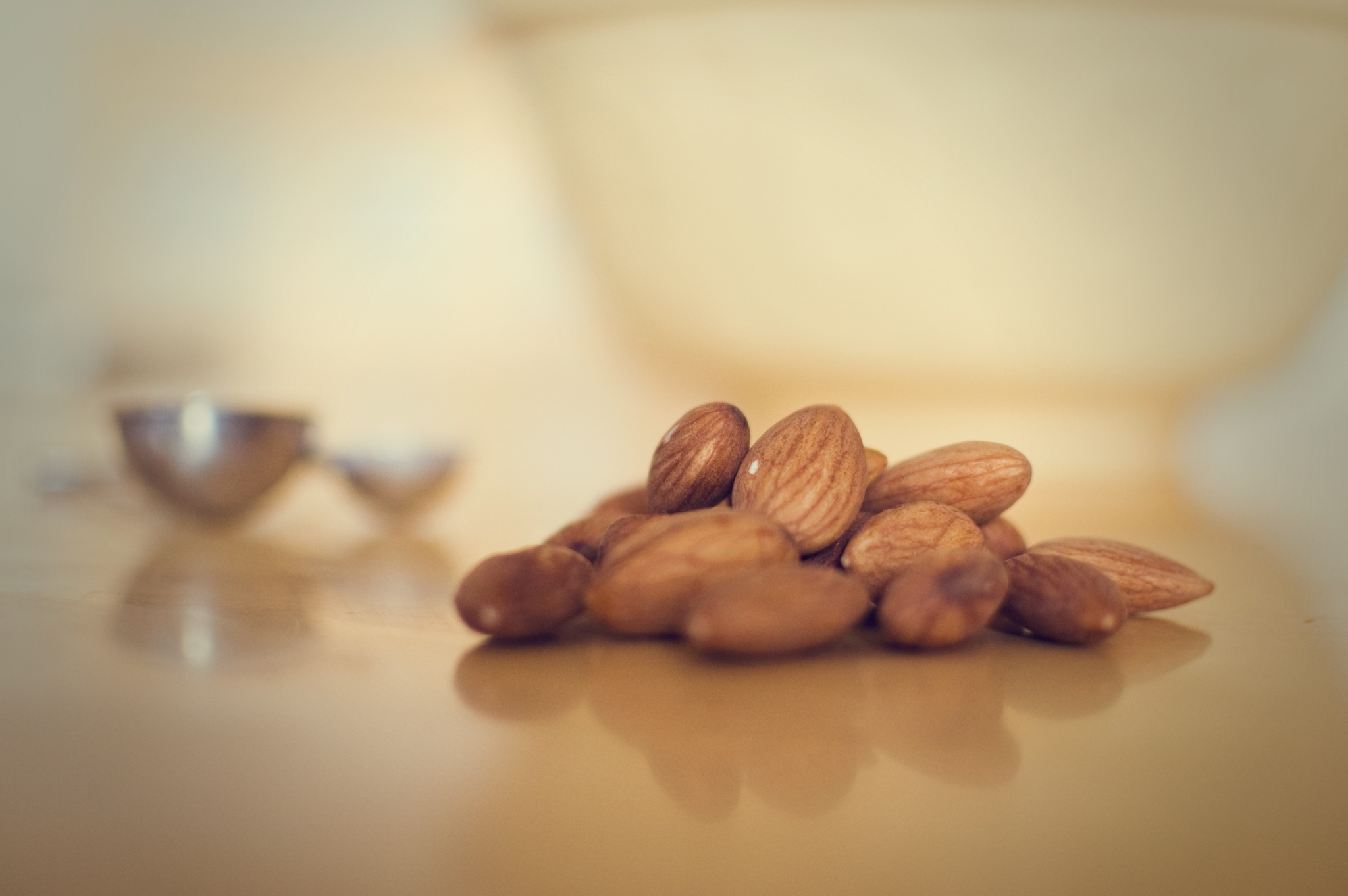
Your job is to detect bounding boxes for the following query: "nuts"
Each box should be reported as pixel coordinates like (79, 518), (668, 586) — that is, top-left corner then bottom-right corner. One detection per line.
(447, 400), (1217, 654)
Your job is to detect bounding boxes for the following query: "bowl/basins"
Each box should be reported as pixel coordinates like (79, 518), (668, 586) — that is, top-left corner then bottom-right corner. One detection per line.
(333, 447), (462, 520)
(114, 407), (309, 521)
